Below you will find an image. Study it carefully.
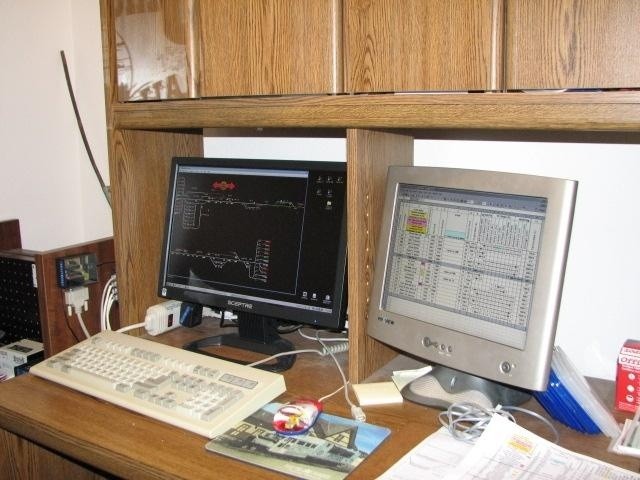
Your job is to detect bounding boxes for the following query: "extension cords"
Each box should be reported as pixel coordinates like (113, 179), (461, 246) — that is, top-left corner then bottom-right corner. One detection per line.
(143, 299), (183, 337)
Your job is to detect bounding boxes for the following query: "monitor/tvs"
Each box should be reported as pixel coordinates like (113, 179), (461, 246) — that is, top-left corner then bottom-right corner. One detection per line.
(364, 165), (579, 415)
(156, 155), (349, 373)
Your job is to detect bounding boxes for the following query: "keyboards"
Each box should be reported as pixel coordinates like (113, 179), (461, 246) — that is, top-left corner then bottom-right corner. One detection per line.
(28, 328), (287, 440)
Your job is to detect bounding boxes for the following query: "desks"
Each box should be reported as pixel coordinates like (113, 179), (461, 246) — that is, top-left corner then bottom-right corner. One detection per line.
(0, 307), (639, 477)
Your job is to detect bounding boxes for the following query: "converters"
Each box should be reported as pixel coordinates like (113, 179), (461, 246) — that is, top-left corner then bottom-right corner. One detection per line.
(180, 302), (202, 326)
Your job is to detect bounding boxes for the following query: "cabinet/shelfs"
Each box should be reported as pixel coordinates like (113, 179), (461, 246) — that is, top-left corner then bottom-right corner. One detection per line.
(0, 215), (117, 356)
(102, 0), (640, 130)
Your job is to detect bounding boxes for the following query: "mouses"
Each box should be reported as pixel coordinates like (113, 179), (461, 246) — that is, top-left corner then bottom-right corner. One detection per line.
(272, 398), (322, 435)
